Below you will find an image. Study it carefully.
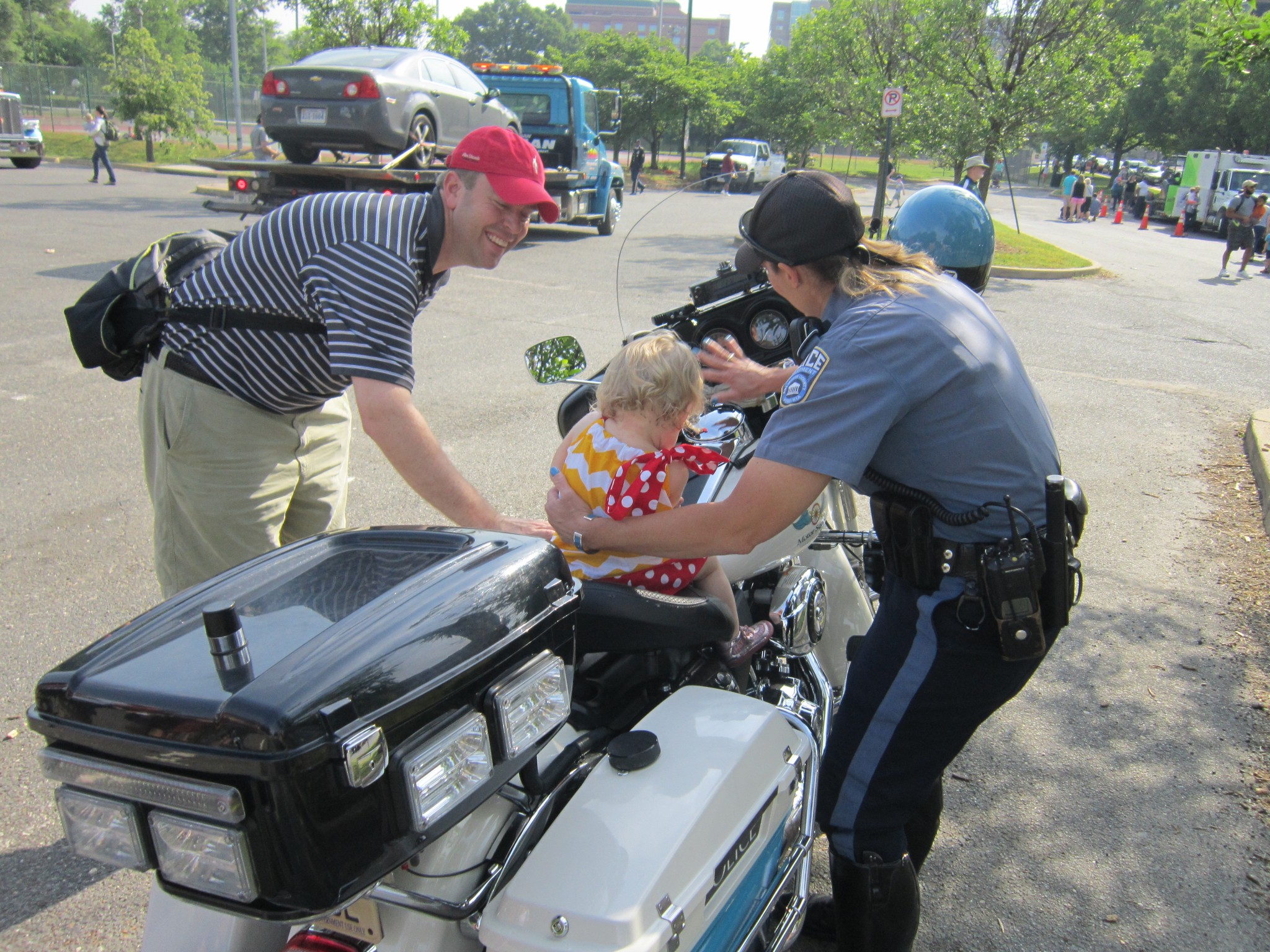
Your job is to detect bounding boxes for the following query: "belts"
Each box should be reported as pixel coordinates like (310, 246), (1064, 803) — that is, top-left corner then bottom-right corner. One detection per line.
(932, 529), (1046, 576)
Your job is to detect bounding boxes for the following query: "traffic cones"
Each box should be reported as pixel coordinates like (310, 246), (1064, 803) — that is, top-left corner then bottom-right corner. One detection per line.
(1111, 201), (1124, 224)
(1137, 205), (1150, 231)
(1169, 210), (1189, 237)
(1096, 189), (1108, 218)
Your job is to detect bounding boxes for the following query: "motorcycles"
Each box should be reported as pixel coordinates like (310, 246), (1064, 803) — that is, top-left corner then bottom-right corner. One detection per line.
(27, 168), (880, 952)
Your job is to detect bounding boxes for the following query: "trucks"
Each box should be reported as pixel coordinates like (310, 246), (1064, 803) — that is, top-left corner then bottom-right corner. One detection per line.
(1163, 149), (1270, 252)
(0, 85), (44, 169)
(190, 62), (625, 237)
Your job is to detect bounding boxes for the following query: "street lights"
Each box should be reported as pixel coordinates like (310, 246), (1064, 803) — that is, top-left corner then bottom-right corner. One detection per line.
(110, 28), (119, 100)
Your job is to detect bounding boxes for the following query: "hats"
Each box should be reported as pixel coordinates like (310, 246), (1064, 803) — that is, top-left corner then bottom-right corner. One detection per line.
(895, 175), (902, 179)
(961, 155), (990, 171)
(734, 171), (865, 275)
(1242, 179), (1258, 188)
(443, 126), (560, 224)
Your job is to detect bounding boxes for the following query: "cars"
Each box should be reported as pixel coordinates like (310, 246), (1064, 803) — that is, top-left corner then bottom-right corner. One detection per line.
(1073, 155), (1182, 180)
(260, 47), (522, 170)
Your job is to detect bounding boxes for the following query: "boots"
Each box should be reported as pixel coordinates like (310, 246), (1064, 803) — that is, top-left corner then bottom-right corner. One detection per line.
(775, 774), (941, 940)
(826, 848), (919, 952)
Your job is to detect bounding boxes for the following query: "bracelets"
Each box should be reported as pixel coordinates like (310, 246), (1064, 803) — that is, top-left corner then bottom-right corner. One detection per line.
(572, 514), (603, 554)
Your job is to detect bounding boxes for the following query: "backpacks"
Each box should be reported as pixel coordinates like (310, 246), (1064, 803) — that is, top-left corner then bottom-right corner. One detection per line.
(97, 118), (118, 141)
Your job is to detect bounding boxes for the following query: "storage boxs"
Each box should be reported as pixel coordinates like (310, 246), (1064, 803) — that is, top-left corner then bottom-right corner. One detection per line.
(26, 525), (585, 918)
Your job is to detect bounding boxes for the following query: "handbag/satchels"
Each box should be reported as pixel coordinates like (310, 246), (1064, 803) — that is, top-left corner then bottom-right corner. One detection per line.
(66, 227), (238, 383)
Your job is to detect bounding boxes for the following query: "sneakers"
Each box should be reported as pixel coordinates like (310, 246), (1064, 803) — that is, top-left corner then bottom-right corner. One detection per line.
(720, 190), (730, 196)
(725, 621), (774, 670)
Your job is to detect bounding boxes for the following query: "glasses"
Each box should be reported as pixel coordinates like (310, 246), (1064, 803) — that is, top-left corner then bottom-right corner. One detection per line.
(739, 209), (794, 267)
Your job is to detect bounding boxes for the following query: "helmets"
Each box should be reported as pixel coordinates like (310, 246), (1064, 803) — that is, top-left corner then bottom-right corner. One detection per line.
(889, 183), (994, 294)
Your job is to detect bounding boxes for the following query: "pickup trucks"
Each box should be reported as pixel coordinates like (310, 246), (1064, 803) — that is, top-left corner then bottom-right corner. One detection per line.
(699, 138), (787, 195)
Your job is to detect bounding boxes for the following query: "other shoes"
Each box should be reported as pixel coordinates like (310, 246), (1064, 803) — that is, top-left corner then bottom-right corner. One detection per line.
(88, 178), (98, 184)
(630, 192), (635, 195)
(640, 186), (645, 194)
(104, 180), (116, 185)
(1236, 270), (1254, 280)
(1218, 270), (1230, 278)
(1260, 268), (1270, 273)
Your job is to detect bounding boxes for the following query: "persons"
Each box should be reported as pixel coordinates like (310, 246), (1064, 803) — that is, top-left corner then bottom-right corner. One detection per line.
(542, 171), (1089, 952)
(993, 154), (1270, 280)
(883, 157), (907, 210)
(955, 156), (990, 207)
(721, 149), (737, 197)
(551, 329), (773, 670)
(250, 113), (278, 181)
(63, 124), (561, 602)
(626, 138), (647, 196)
(81, 105), (120, 186)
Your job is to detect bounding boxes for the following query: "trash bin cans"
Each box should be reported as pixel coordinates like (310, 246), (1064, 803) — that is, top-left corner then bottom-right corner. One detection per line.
(1050, 172), (1064, 189)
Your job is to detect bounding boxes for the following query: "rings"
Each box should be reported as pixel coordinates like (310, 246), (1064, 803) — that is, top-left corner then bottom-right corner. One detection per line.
(726, 352), (735, 362)
